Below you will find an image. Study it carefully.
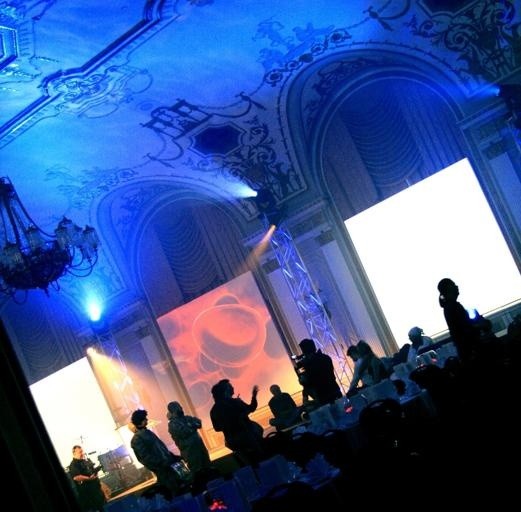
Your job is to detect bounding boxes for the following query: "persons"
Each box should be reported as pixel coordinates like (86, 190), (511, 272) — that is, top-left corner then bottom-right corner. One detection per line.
(299, 338), (343, 406)
(130, 409), (185, 490)
(209, 380), (263, 456)
(356, 339), (388, 383)
(407, 328), (434, 362)
(166, 400), (211, 472)
(437, 276), (475, 354)
(346, 343), (374, 389)
(267, 385), (304, 432)
(69, 445), (106, 512)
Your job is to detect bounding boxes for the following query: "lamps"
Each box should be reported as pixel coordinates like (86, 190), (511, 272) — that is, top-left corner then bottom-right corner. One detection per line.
(496, 84), (521, 128)
(267, 205), (286, 227)
(253, 189), (275, 210)
(0, 175), (99, 305)
